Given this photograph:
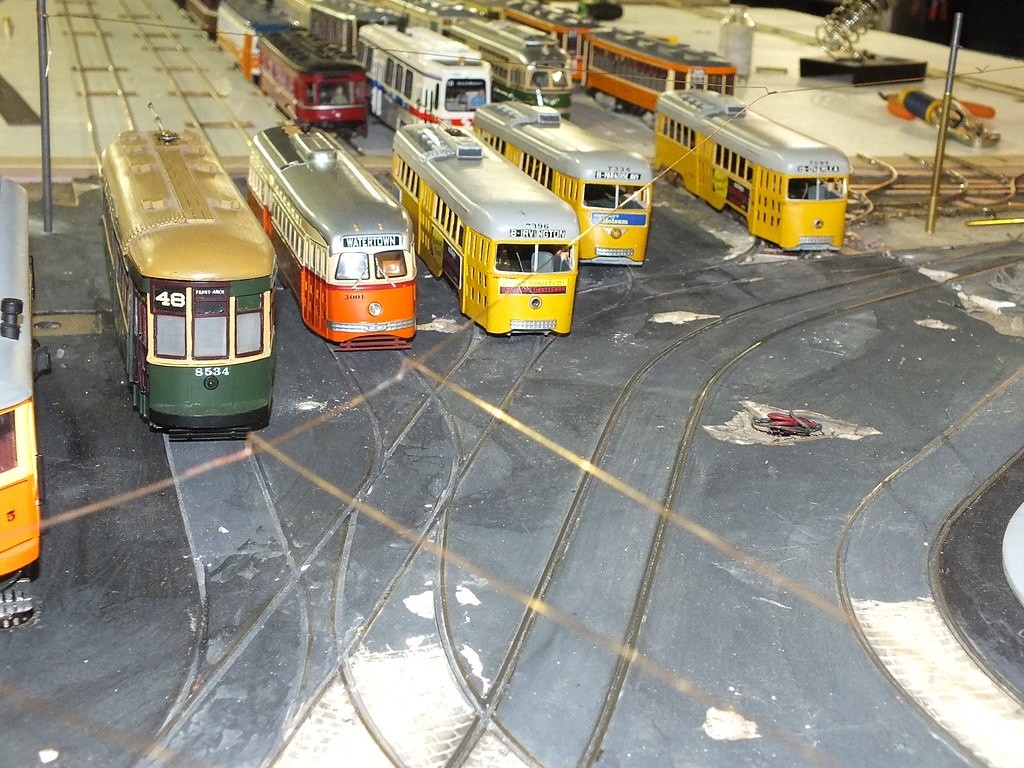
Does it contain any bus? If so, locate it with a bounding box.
[356,24,491,134]
[580,29,736,122]
[100,129,276,445]
[451,20,572,120]
[655,88,851,252]
[185,0,218,31]
[309,1,408,55]
[248,125,415,350]
[466,0,503,21]
[500,0,600,77]
[473,102,653,266]
[260,29,369,140]
[0,173,40,581]
[380,1,484,34]
[219,1,301,84]
[391,117,579,336]
